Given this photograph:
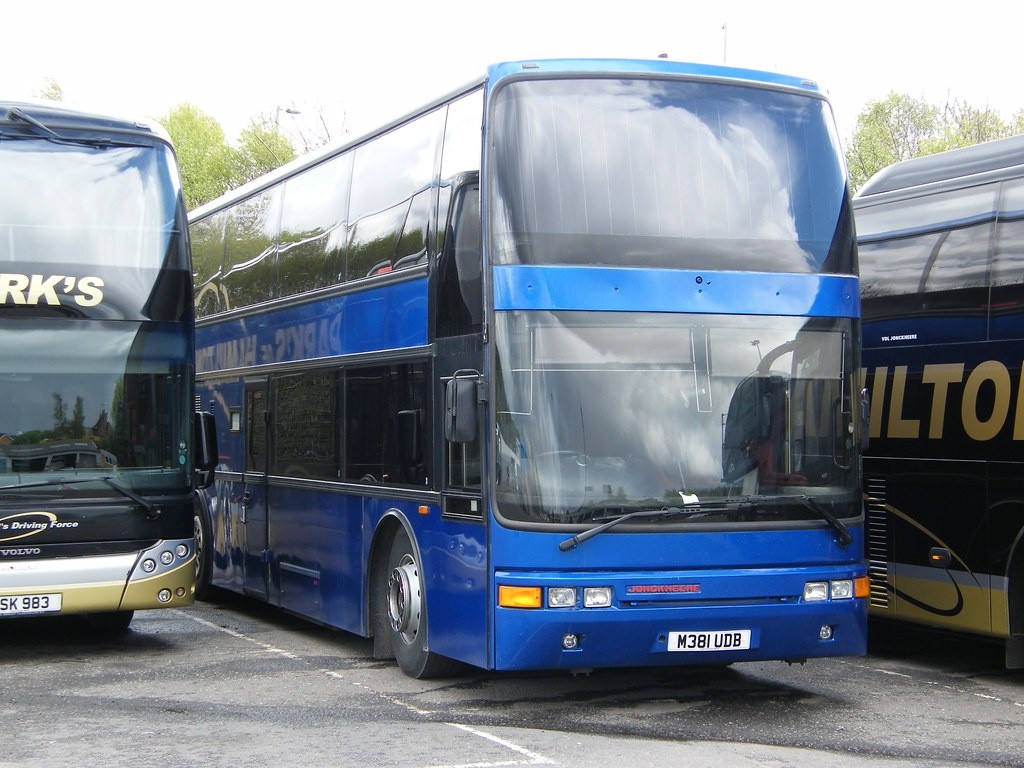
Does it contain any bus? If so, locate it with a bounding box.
[0,96,220,646]
[183,53,871,681]
[852,133,1024,671]
